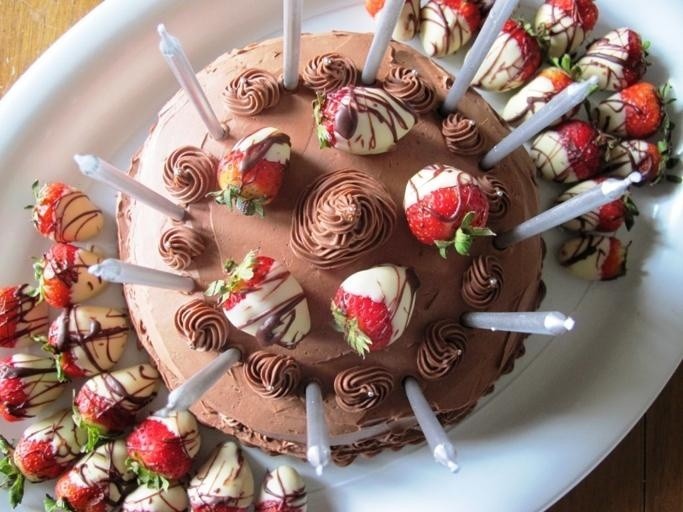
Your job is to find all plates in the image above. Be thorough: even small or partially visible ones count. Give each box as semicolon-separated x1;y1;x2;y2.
0;0;681;512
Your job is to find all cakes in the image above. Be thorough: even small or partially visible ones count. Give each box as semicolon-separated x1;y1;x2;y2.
70;0;645;472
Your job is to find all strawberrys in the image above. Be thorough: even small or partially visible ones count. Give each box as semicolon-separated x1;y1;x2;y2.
361;1;680;283
0;179;310;512
